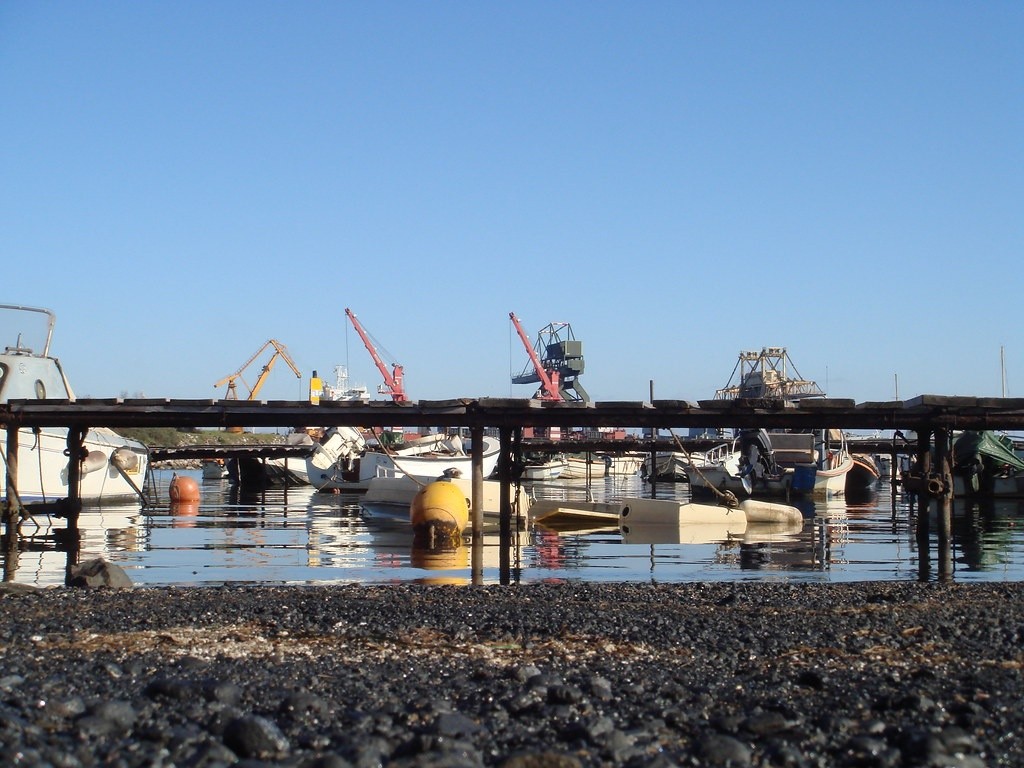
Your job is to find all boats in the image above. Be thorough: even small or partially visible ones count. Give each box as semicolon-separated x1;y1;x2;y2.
216;345;1024;582
0;304;151;501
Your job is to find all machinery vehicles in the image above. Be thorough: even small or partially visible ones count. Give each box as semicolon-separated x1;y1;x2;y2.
212;339;302;435
477;304;631;441
337;306;425;444
